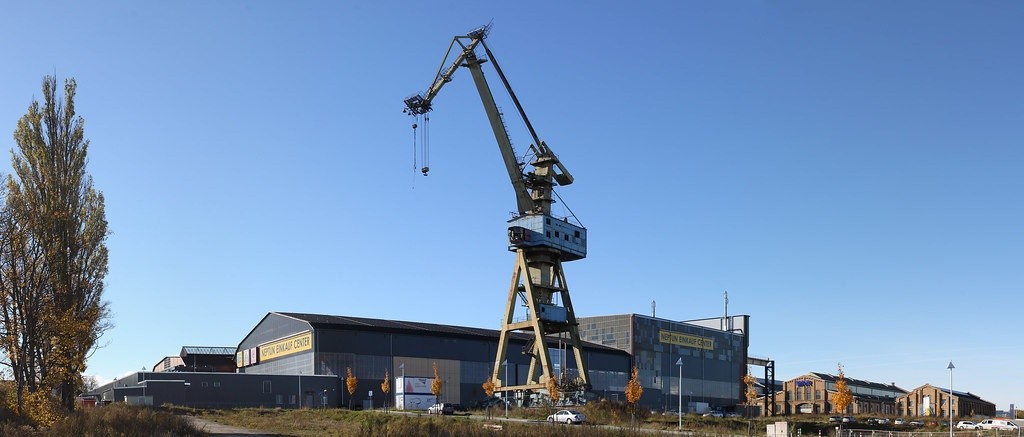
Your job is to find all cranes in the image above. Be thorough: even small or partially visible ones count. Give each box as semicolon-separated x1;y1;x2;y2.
403;14;594;396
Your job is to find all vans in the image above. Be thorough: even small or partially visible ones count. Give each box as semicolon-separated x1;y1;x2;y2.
991;420;1019;432
978;419;993;430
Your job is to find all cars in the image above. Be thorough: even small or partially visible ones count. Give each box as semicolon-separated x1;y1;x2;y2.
428;403;454;415
910;419;923;427
957;421;980;431
867;417;878;425
547;409;586;424
877;418;887;425
894;418;906;425
940;422;949;428
842;416;856;423
829;416;842;423
929;422;939;427
